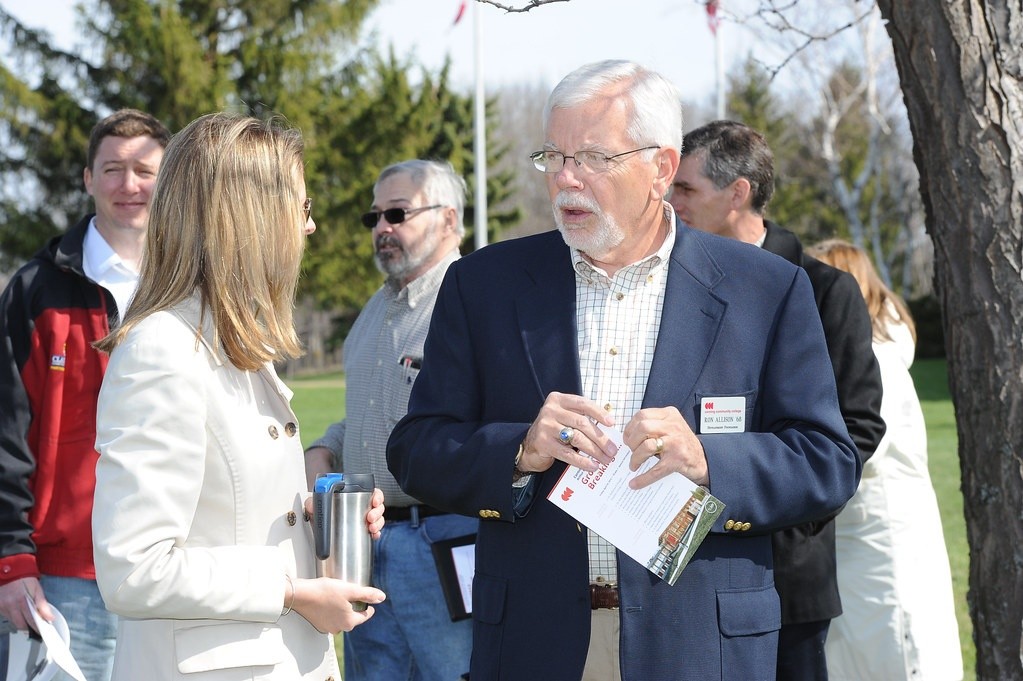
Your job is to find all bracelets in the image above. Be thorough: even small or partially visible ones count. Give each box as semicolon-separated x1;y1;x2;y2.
281;573;294;617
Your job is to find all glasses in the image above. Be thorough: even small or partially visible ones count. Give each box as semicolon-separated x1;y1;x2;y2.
361;204;445;227
303;197;312;222
528;146;660;172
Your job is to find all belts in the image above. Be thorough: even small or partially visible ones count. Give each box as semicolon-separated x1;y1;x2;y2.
382;504;449;522
590;584;619;610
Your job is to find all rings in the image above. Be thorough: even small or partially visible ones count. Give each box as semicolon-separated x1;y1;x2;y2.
652;436;665;454
559;426;575;445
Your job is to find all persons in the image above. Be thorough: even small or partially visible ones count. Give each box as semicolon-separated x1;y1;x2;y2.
92;108;386;681
387;61;861;681
797;235;966;681
303;156;479;681
670;120;887;681
0;107;177;681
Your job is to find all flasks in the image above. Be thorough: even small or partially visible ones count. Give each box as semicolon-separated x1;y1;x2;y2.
313;472;375;613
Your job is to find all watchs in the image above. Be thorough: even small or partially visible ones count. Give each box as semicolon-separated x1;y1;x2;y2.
514;439;537;478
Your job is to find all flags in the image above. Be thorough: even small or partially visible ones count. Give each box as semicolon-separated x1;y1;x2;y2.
704;0;723;34
449;1;473;29
